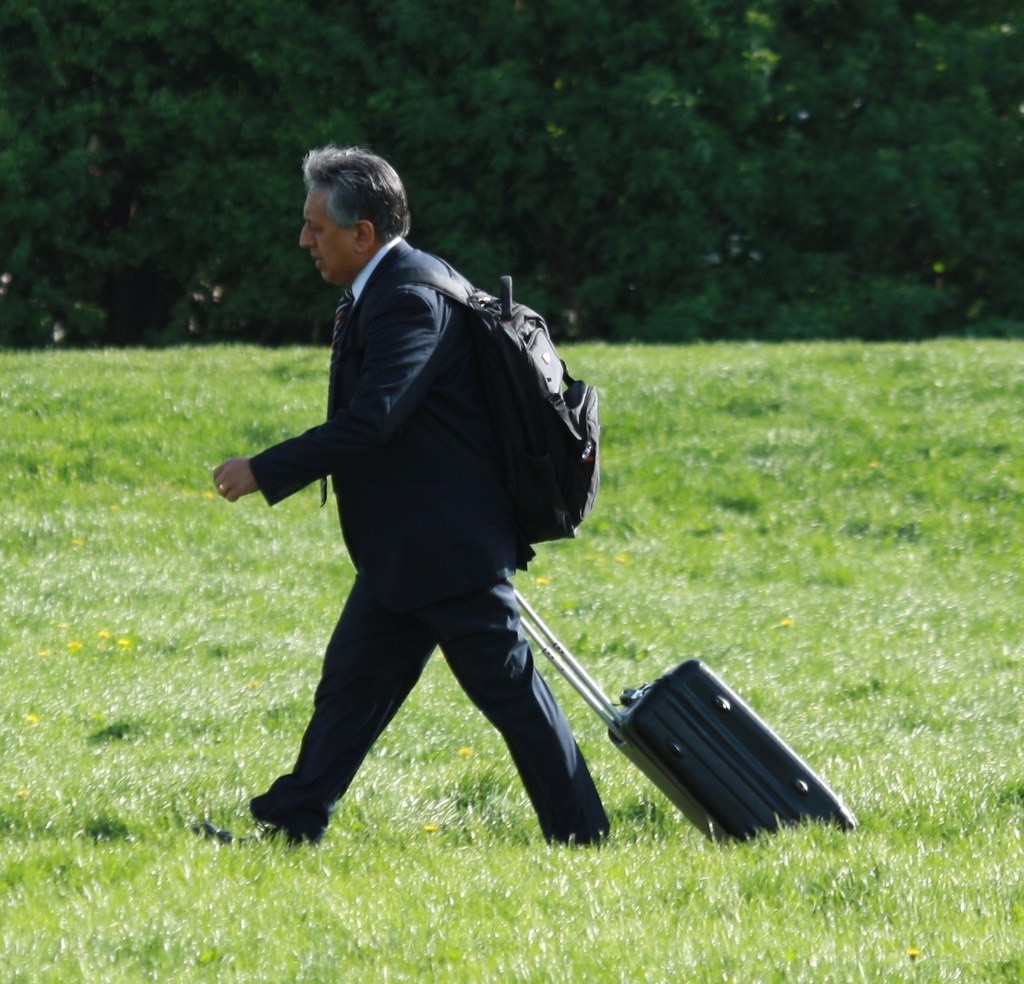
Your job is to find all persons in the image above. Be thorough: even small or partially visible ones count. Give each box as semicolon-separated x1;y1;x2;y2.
193;147;610;851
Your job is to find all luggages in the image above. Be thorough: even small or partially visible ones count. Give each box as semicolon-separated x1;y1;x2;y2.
499;573;856;848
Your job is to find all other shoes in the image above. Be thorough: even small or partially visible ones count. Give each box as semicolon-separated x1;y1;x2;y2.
192;814;284;851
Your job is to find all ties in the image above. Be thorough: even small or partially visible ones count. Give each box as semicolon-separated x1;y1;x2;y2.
316;289;354;508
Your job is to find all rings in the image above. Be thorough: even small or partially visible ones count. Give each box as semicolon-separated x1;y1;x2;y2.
219;483;227;493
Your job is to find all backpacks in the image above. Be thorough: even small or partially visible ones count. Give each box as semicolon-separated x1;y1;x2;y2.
349;255;603;543
